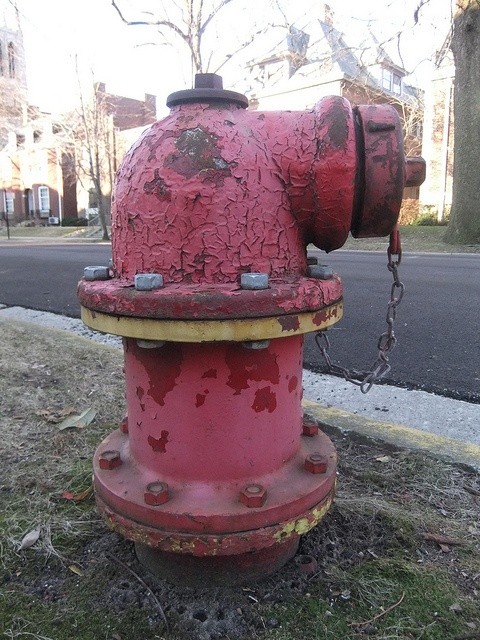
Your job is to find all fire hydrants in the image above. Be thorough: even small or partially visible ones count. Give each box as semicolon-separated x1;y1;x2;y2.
74;71;427;588
